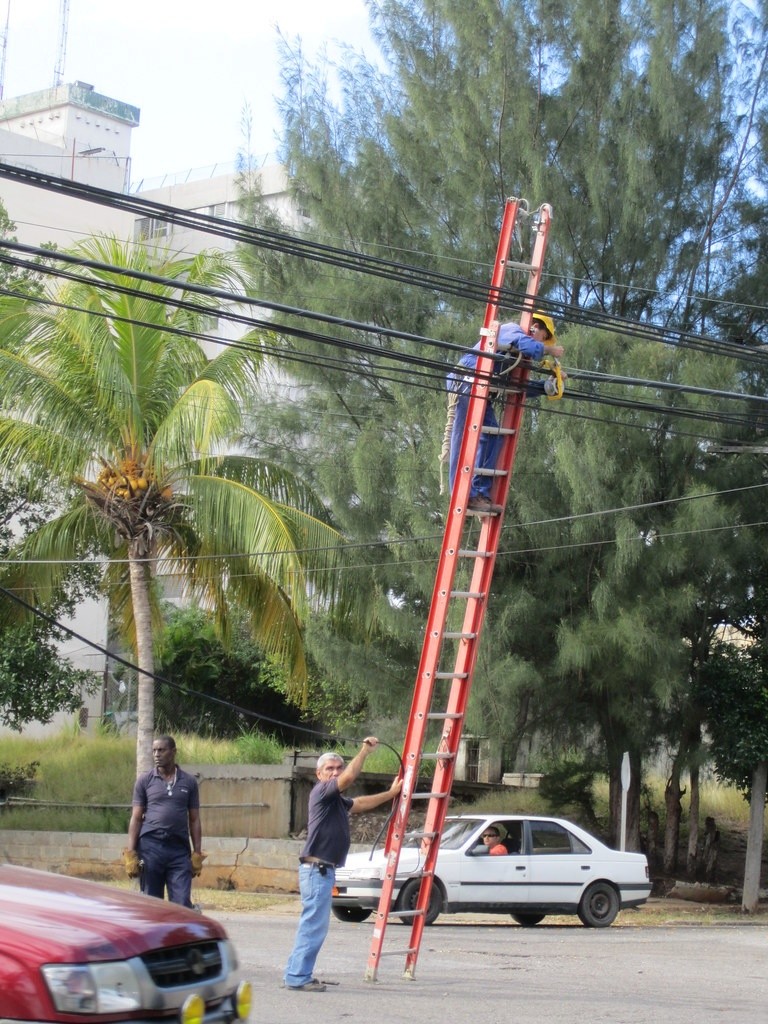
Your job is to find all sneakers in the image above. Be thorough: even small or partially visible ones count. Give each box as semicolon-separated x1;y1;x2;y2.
282;978;327;992
467;497;504;514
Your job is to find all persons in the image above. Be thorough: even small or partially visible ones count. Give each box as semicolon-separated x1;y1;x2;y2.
445;308;568;513
480;826;509;855
119;734;209;911
279;736;404;992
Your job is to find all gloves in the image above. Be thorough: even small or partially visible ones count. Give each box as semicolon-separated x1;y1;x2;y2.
125;847;141;879
191;851;208;878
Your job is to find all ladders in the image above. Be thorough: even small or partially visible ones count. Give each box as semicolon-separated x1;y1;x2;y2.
362;195;555;982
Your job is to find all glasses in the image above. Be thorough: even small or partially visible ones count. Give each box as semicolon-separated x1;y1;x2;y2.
482;833;498;838
166;783;173;797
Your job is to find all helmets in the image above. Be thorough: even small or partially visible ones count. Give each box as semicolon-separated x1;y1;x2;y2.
532;309;557;346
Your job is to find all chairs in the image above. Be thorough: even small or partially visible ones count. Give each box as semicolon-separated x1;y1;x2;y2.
501;826;521;852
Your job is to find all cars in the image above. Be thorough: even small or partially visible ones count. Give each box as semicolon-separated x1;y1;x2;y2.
1;862;253;1024
331;815;654;928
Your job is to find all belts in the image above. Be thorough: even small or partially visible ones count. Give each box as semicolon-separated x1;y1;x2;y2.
298;856;334;868
